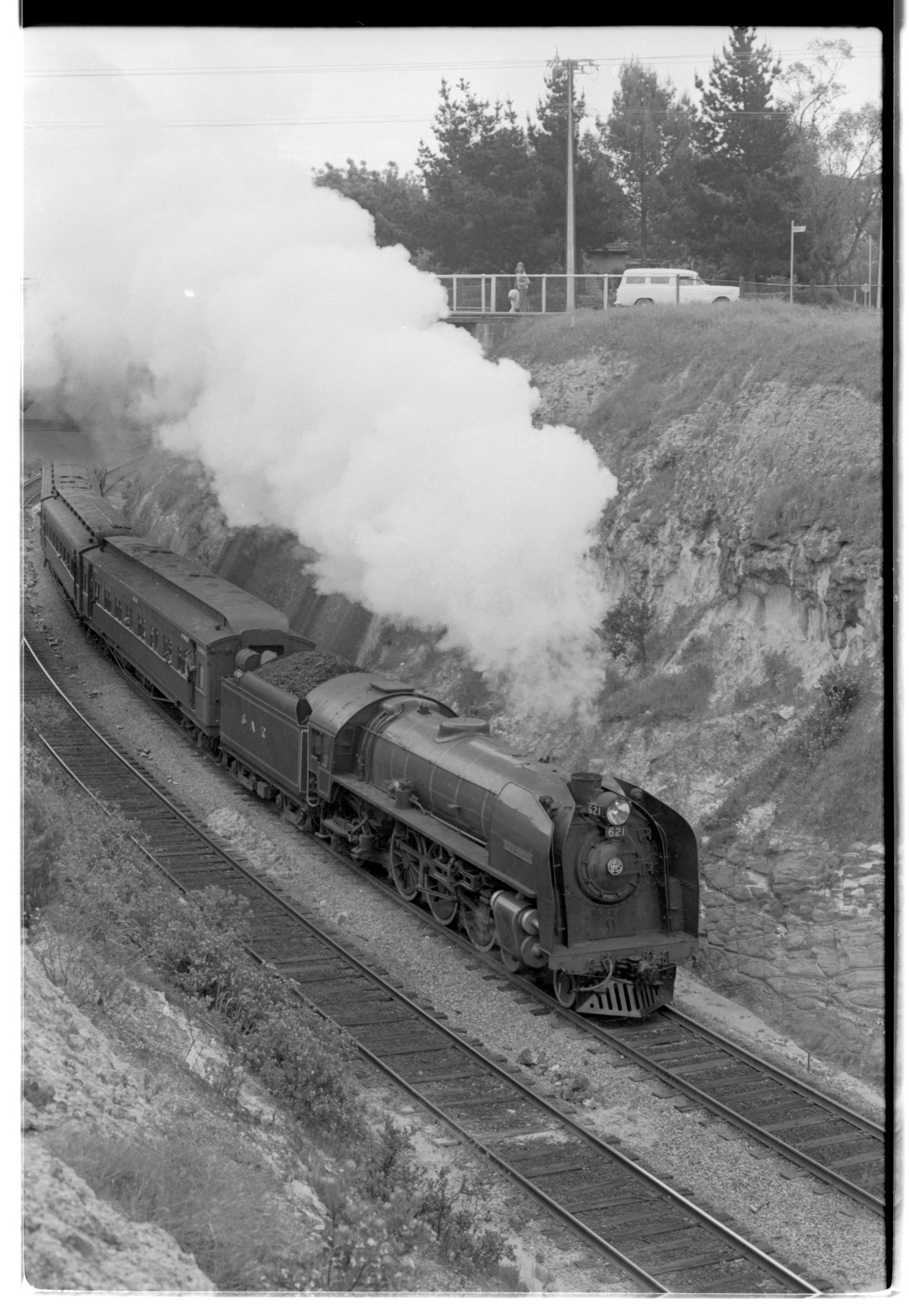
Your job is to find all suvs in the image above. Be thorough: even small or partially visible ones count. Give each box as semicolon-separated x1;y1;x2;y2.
614;268;740;310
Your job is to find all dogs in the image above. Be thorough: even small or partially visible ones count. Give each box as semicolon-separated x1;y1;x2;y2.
508;289;533;312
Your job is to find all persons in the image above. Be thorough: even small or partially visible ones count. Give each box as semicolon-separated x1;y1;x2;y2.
515;262;530;312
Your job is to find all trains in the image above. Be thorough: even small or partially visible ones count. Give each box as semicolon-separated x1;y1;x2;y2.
39;460;707;1025
